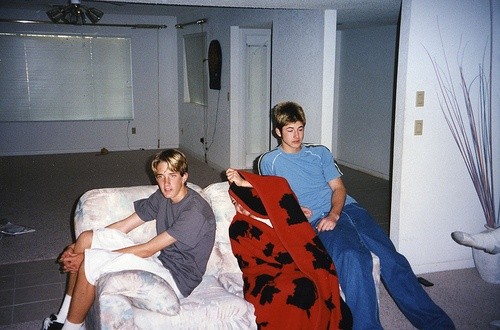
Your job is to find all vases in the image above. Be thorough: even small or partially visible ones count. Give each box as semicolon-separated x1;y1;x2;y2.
472;225;500;285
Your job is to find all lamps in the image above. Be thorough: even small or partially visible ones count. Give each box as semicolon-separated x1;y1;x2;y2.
47;0;104;25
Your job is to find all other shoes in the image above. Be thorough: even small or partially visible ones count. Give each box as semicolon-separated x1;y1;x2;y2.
42;314;64;330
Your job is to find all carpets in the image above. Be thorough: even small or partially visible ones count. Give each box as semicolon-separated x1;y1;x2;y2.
0;149;229;265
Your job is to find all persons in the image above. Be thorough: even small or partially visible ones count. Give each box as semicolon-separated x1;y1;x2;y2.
226;168;355;330
42;149;217;330
259;101;456;330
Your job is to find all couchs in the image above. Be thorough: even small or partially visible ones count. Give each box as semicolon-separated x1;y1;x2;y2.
73;182;380;330
